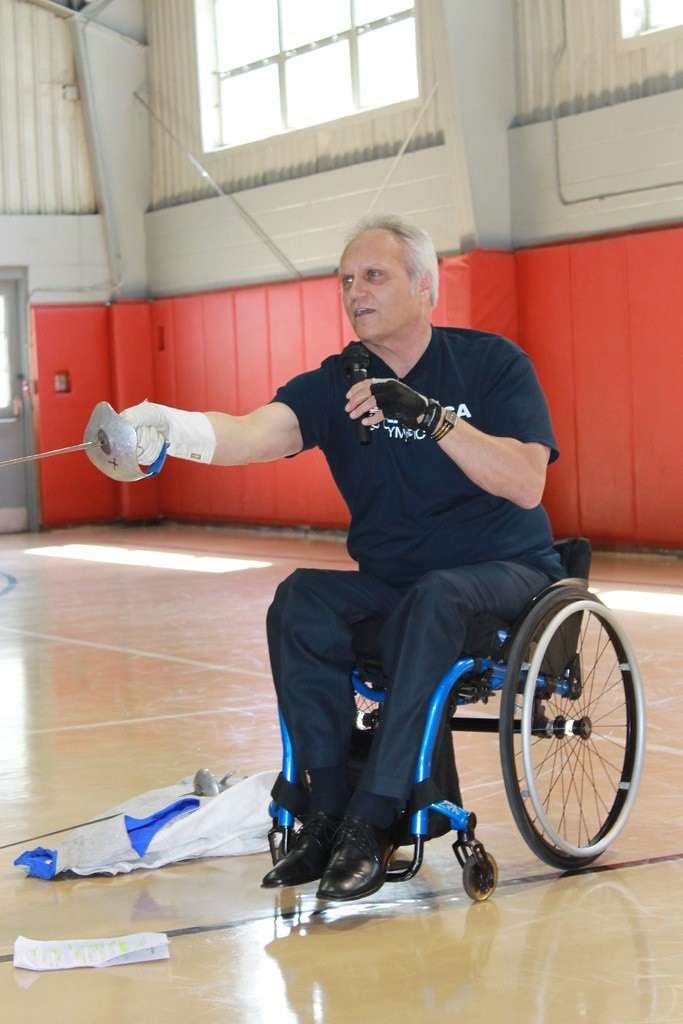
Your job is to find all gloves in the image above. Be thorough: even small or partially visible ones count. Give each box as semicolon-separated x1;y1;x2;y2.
119;403;169;465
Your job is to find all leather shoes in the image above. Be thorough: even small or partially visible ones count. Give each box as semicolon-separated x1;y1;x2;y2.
316;810;409;901
260;809;339;888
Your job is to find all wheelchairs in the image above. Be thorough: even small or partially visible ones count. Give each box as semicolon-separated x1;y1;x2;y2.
266;534;646;903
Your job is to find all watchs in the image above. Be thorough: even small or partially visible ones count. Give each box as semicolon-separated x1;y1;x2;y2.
431;408;458;442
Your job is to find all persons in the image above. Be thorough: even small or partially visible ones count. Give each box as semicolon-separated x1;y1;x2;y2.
120;211;566;901
